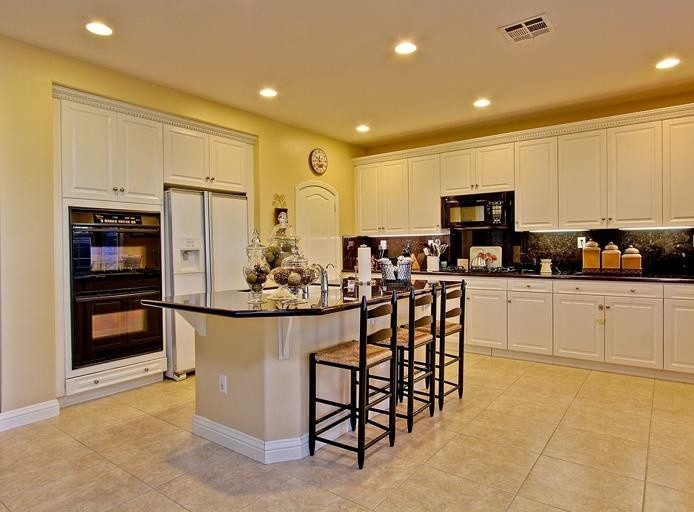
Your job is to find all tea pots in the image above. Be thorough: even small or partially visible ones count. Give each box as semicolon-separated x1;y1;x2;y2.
471;248;490;268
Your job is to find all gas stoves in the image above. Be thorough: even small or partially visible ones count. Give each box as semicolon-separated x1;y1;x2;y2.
441;264;523;276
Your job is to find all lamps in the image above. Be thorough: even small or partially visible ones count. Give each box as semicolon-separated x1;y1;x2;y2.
309;149;328;175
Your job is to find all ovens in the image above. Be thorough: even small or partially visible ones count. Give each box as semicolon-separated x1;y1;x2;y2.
70;290;165;368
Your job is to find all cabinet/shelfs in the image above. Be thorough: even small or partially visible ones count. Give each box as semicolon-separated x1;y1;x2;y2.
557;118;662;229
662;115;693;229
553;279;663;371
353;158;407;239
60;99;163;205
662;282;694;376
515;135;558;232
343;271;507;351
163;124;247;194
406;152;448;237
440;142;515;192
508;277;553;357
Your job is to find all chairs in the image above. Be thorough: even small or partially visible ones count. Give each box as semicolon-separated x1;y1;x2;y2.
310;280;466;470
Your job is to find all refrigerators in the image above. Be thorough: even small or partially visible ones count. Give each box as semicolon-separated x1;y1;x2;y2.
165;186;250;381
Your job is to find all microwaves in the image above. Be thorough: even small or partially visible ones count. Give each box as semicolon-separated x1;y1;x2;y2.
441;197;507;227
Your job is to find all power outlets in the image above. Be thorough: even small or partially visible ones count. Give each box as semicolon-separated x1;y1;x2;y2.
577;237;586;248
218;374;228;393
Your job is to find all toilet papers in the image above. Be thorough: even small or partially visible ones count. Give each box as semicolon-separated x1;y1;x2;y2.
359;286;371;301
358;247;371;281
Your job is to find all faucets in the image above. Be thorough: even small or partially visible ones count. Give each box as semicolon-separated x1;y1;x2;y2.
326;263;342;278
